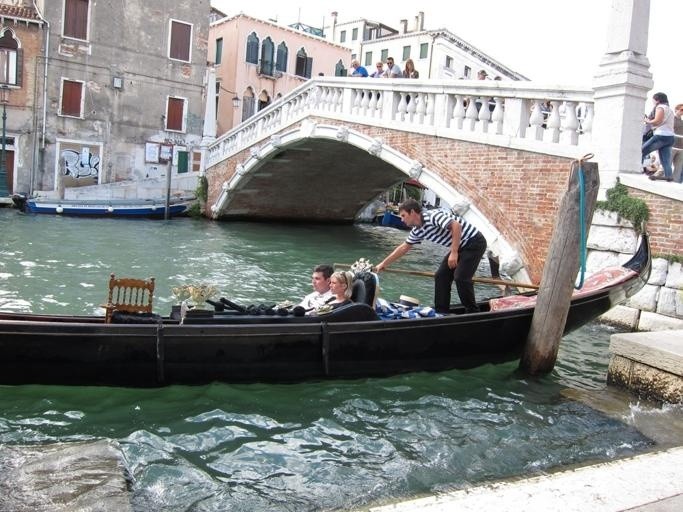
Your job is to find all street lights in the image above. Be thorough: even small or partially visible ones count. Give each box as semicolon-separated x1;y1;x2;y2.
0;82;9;197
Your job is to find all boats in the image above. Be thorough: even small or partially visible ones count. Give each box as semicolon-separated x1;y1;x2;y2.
10;193;187;219
374;203;411;230
0;219;653;389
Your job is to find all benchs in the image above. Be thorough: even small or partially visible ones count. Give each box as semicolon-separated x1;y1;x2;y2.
346;272;383;309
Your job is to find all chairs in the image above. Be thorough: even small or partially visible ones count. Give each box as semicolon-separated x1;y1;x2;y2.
99;272;155;323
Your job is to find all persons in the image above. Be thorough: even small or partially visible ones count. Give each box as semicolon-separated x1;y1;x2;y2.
402;59;419;113
324;271;353;310
351;59;368;77
319;73;324;76
297;265;336;315
379;57;402;112
473;70;552;129
642;92;683;184
375;199;487;314
369;62;384;78
